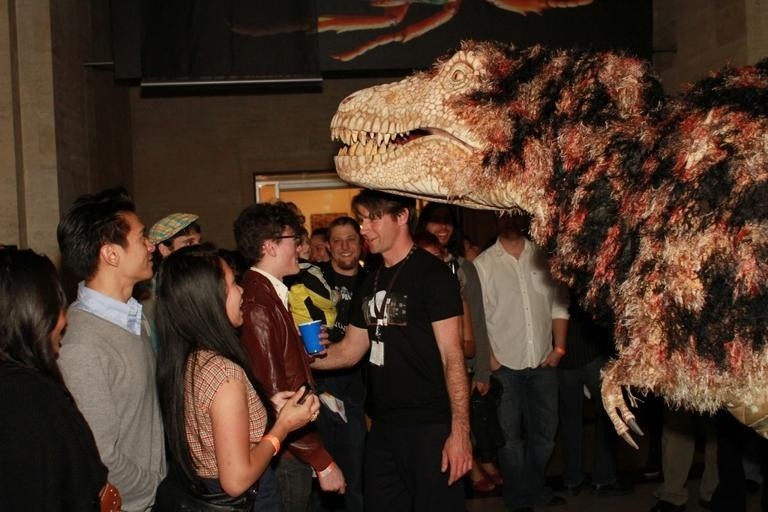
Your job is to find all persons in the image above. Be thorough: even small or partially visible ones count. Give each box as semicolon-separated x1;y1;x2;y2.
58;185;767;511
0;244;125;511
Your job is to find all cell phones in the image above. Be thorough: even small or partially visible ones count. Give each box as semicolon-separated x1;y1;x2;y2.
292;380;313;404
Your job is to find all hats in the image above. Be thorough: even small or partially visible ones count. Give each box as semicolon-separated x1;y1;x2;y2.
148;212;200;245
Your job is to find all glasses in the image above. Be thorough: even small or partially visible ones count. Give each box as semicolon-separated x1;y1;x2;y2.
260;232;302;246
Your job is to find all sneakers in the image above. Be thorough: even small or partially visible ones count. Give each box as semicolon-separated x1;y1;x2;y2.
649;499;688;512
698;497;712;509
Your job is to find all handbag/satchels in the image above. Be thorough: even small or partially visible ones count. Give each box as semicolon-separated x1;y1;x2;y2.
174;484;255;511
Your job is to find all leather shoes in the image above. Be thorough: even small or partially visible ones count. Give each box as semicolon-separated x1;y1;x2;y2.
463;469;505;493
542;477;635;509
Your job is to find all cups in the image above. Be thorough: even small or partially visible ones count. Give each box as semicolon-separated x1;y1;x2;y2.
298;319;325;355
